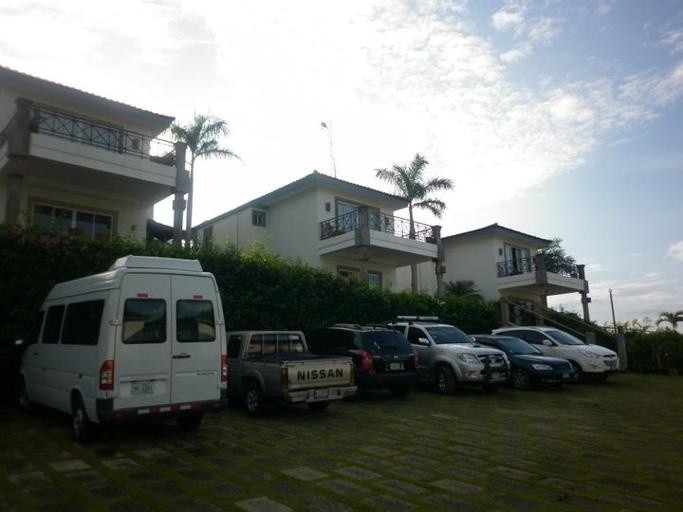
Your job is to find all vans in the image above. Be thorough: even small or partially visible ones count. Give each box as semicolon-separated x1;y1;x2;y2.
15;256;228;443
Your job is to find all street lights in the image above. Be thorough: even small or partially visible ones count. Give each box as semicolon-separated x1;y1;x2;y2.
321;122;336;178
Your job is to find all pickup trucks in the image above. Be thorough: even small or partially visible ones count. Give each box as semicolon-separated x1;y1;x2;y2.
227;325;358;418
